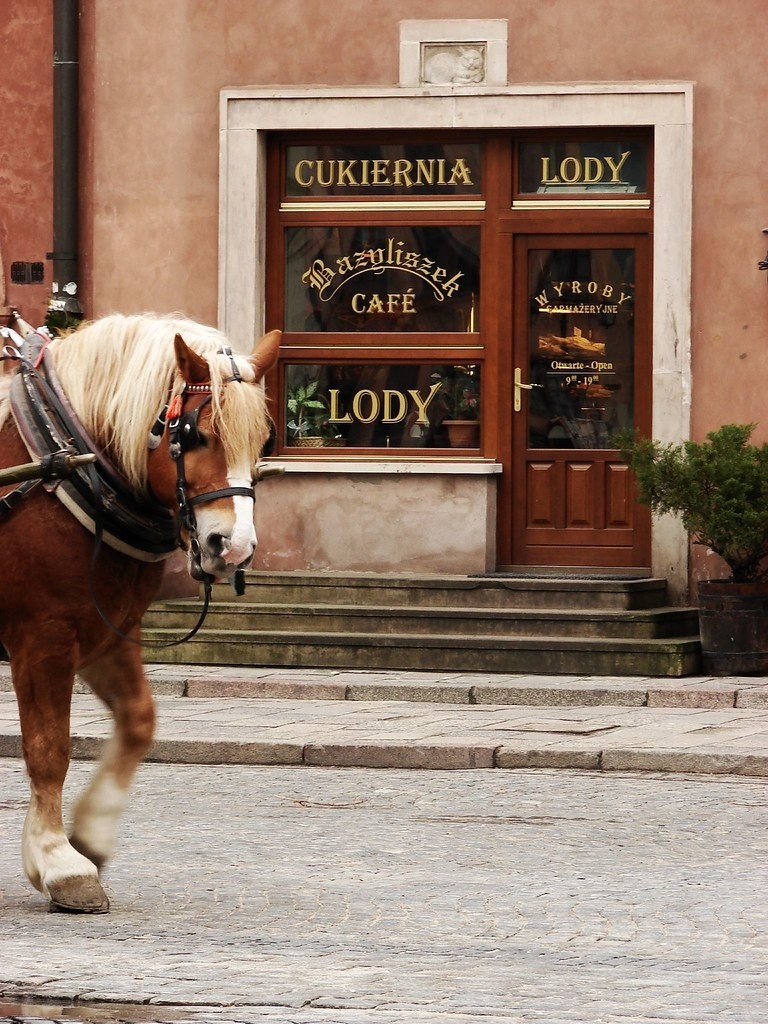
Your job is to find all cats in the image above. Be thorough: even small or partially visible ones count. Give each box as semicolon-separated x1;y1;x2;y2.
424;46;484;84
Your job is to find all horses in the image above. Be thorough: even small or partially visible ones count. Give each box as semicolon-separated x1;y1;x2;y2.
0;312;283;914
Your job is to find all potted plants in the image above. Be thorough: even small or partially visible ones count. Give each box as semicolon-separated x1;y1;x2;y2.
603;421;768;676
287;380;346;447
436;370;480;447
